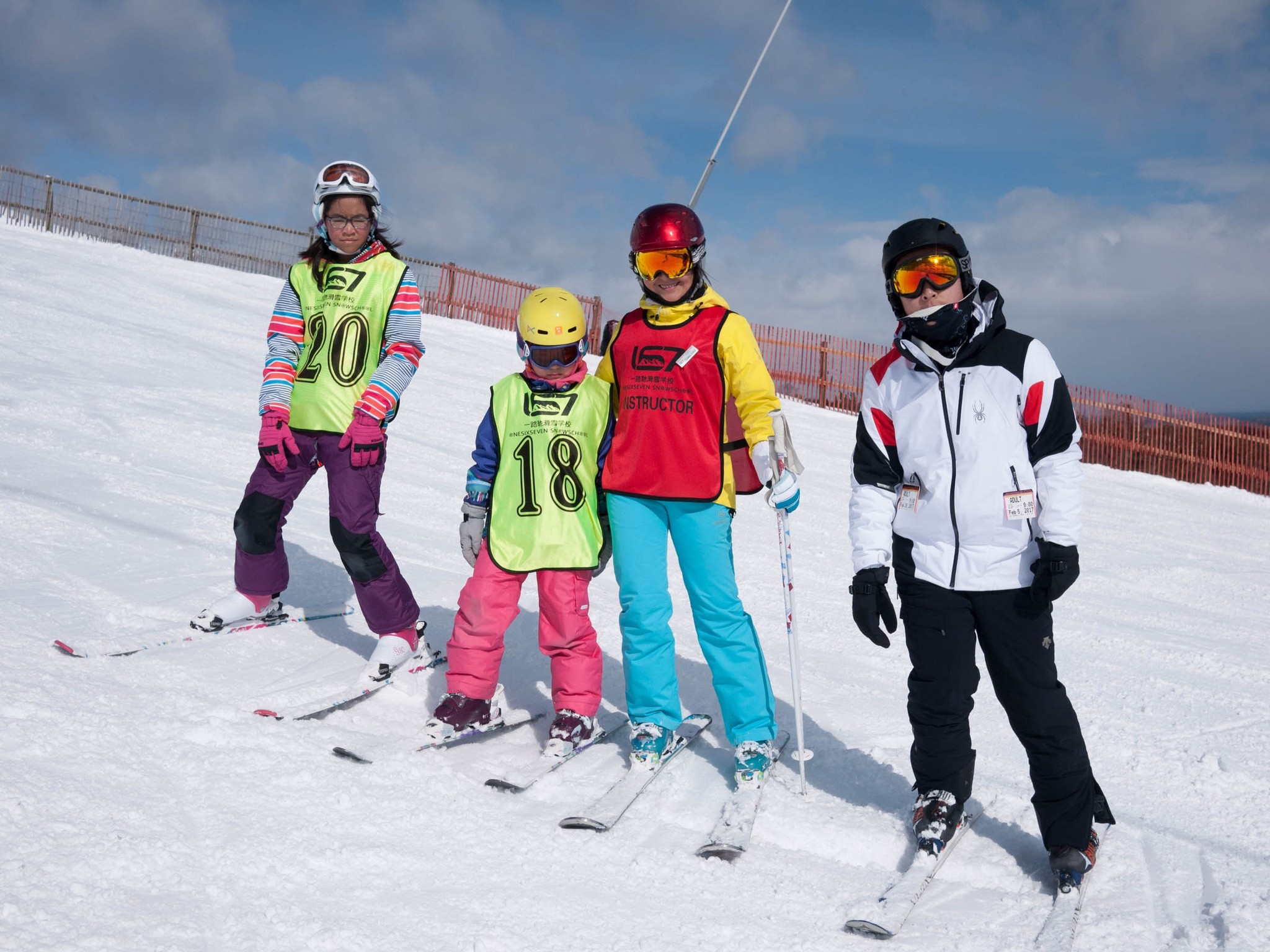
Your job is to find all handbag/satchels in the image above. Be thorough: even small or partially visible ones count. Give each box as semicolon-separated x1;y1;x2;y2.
591;515;612;577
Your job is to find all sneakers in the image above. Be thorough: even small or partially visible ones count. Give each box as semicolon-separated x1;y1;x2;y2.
433;693;492;732
368;621;418;668
910;789;963;846
734;739;771;772
209;589;272;623
629;723;673;760
549;710;594;744
1049;828;1097;878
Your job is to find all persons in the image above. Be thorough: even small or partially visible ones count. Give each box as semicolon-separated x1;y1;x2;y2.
189;160;426;681
848;218;1113;884
433;286;618;757
594;203;803;786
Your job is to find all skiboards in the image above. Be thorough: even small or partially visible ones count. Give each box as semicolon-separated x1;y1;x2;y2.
845;789;1110;951
332;711;628;794
49;601;448;724
559;714;787;860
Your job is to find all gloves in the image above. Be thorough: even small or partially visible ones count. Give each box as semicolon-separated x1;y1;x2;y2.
459;502;486;568
339;407;385;466
257;411;300;472
848;566;897;648
763;466;800;514
1027;537;1080;612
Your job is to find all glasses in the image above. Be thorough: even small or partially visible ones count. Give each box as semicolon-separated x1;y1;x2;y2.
892;252;961;297
325;214;370;229
529;343;579;370
635;247;692;282
318;160;374;191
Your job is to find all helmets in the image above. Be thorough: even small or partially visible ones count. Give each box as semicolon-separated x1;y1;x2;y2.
881;217;972;319
312;166;380;241
516;287;587;366
629;203;705;252
